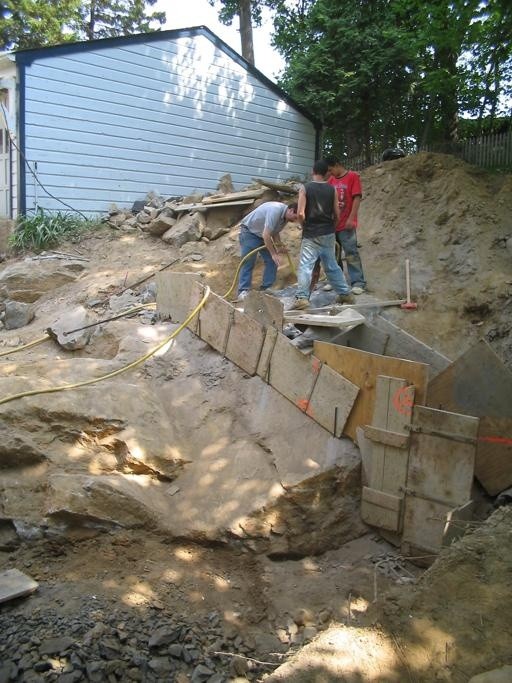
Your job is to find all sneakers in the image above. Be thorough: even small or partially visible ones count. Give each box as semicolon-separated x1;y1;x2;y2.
239;284;365;309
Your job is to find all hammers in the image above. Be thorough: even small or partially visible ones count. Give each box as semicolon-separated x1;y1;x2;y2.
400;259;417;309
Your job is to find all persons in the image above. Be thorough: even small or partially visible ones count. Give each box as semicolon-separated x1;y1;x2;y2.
236;199;298;303
292;159;357;310
322;156;366;295
